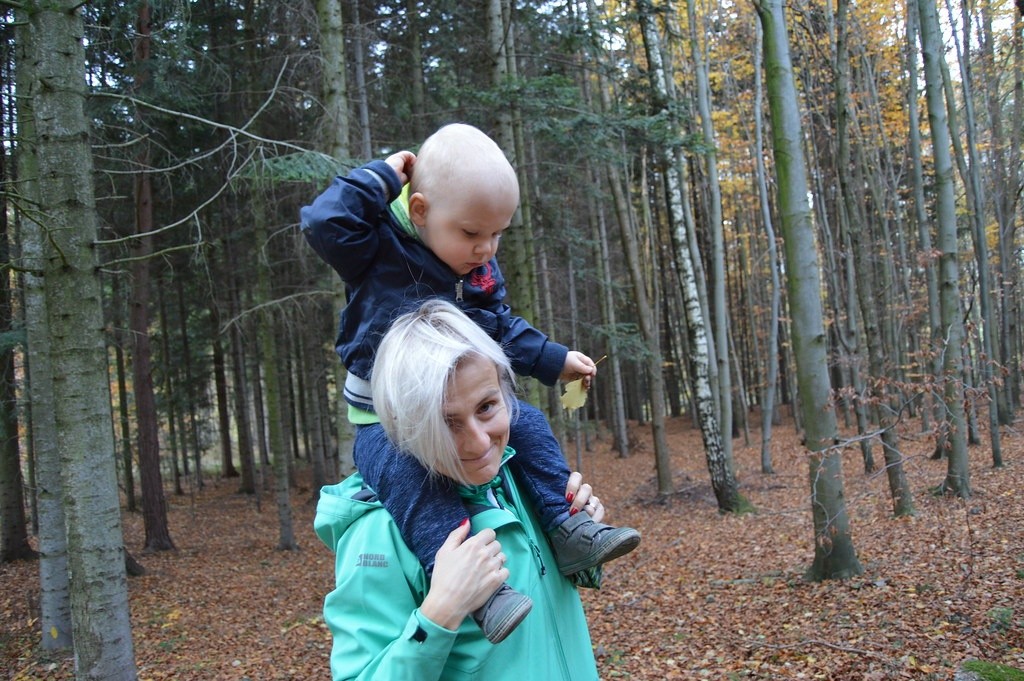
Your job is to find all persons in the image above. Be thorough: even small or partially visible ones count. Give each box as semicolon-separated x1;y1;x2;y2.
299;122;643;646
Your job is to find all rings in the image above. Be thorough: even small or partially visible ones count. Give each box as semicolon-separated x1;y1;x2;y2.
495;555;504;565
313;299;600;681
585;501;598;511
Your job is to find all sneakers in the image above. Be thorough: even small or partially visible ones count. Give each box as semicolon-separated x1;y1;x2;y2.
472;582;534;644
549;510;641;575
